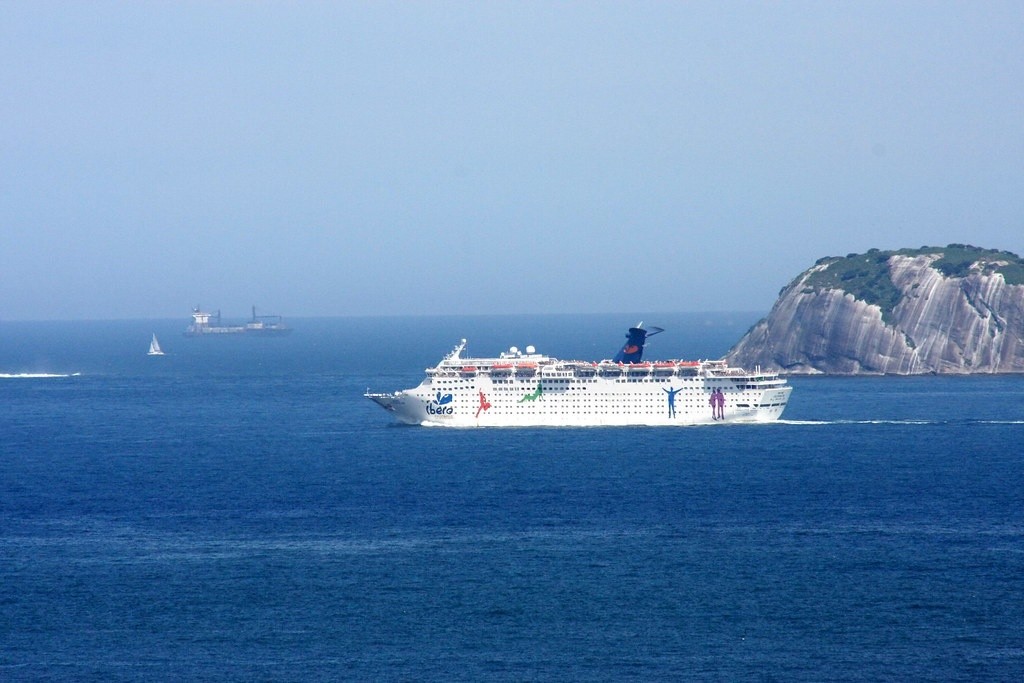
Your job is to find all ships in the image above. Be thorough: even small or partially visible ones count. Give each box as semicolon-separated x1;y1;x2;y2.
363;318;793;430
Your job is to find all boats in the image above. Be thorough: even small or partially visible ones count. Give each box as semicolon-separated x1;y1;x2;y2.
181;304;293;337
628;360;652;372
653;360;677;371
461;365;479;373
491;360;515;372
679;360;699;368
515;360;538;371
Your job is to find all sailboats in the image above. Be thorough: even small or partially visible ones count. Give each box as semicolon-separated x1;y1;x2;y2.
146;331;165;356
